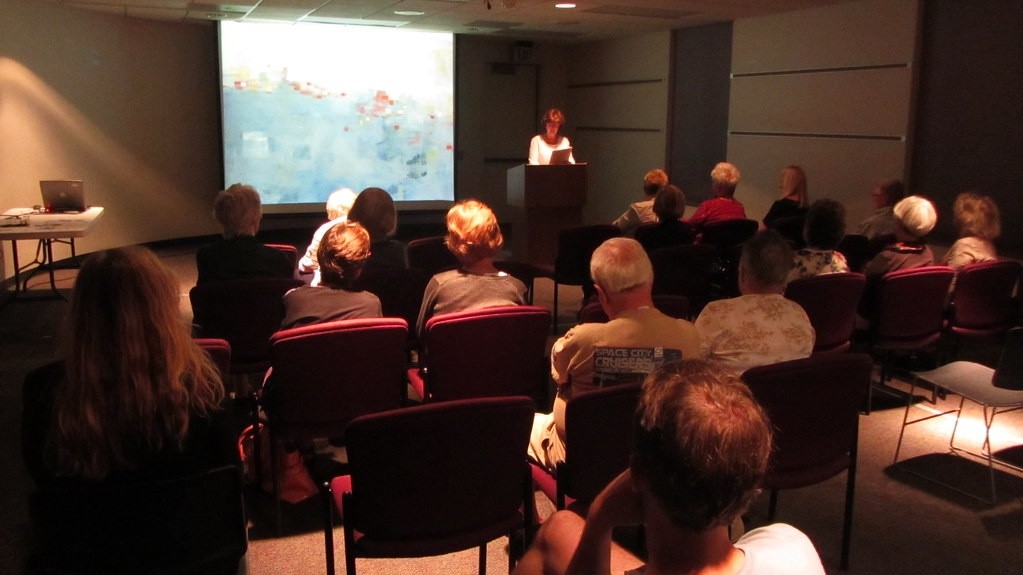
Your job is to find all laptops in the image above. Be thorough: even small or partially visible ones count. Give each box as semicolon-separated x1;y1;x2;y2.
549;147;572;166
39;180;92;212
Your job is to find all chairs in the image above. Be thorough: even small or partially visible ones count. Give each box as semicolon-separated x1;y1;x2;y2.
526;214;804;319
741;338;860;572
530;374;647;517
780;271;866;352
265;244;313;292
861;264;954;415
319;396;538;575
406;236;447;276
236;315;422;542
406;307;555;440
190;337;232;408
492;258;535;308
933;259;1023;394
893;323;1023;506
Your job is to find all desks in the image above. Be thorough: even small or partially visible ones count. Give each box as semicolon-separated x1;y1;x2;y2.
0;205;105;303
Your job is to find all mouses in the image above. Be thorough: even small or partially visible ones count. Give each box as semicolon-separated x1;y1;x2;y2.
33;205;41;210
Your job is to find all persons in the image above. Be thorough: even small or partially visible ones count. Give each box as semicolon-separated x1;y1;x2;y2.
784;181;1003;292
611;161;809;249
189;185;529;493
22;247;247;575
529;107;578;166
510;227;830;575
